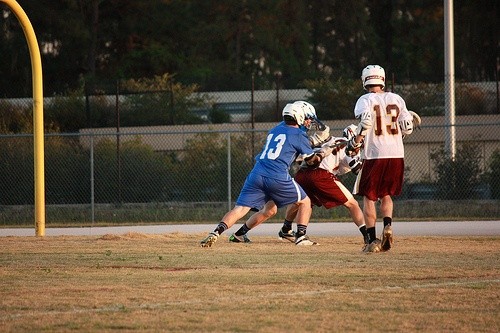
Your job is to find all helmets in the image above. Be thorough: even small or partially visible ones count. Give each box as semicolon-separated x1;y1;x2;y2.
282;103;305;126
344;125;359;140
293;100;316;120
361;65;386;91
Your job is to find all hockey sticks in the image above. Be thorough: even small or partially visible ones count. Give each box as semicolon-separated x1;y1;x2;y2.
313;140;349;153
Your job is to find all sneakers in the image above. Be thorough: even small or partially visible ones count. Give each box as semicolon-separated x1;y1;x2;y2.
201;231;219;248
229;232;250;243
363;239;380;253
381;226;392;251
279;228;297;243
295;235;318;246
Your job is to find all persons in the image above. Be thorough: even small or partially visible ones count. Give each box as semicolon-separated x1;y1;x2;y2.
281;123;366;242
200;101;335;246
345;64;413;253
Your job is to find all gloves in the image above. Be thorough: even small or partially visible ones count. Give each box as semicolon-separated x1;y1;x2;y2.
317;137;337;158
310;125;330;145
345;137;362;158
